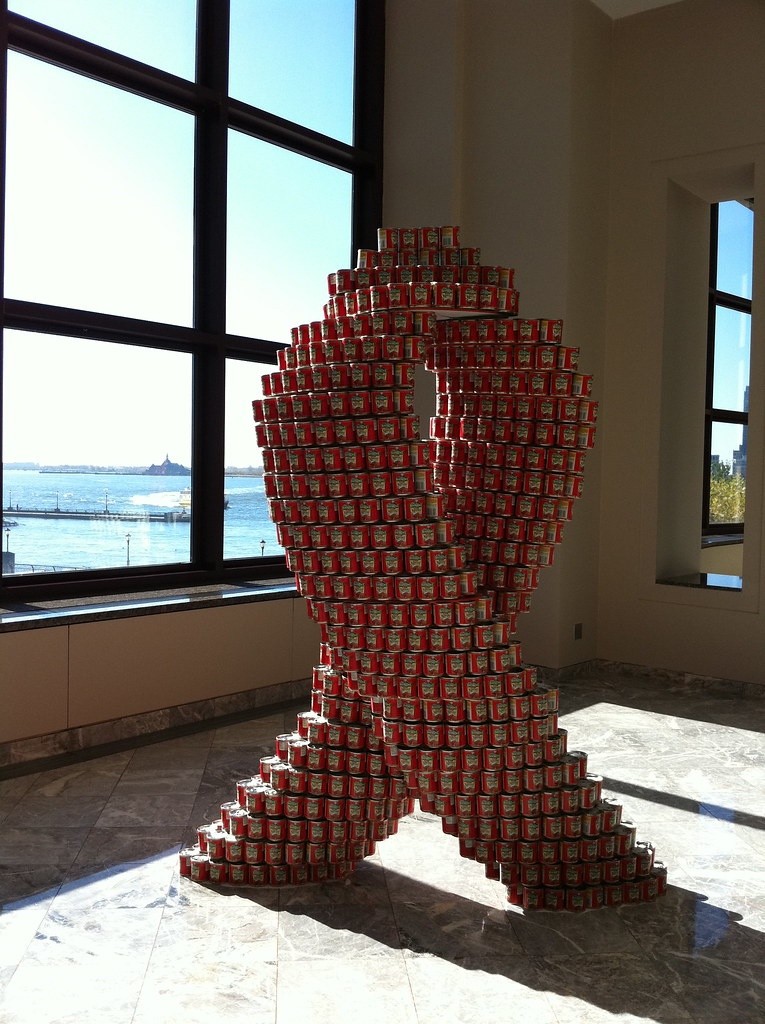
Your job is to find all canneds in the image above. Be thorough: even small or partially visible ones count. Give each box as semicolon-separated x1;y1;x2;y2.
180;226;667;912
